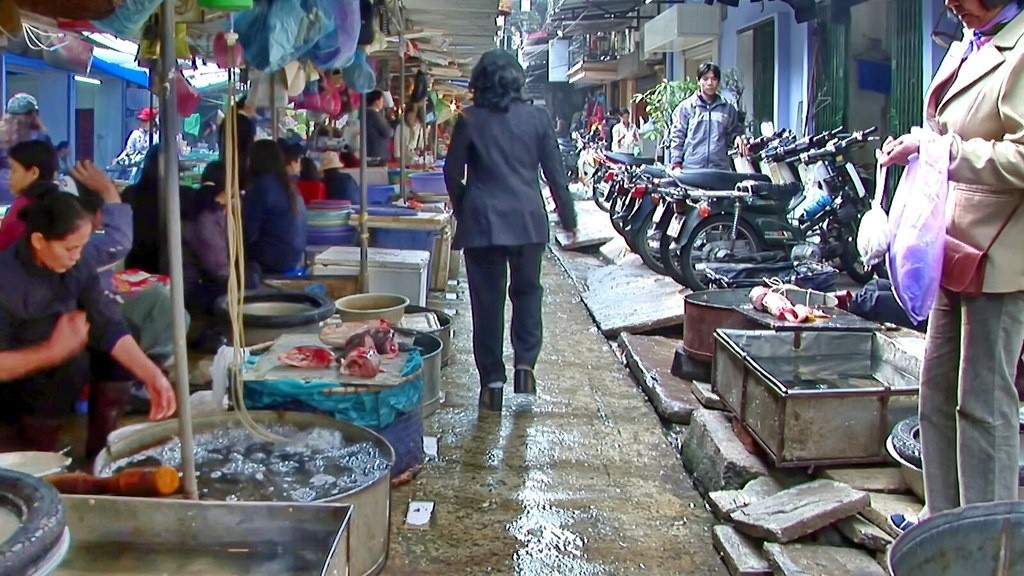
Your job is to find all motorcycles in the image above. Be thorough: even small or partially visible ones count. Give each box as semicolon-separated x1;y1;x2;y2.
538;113;902;295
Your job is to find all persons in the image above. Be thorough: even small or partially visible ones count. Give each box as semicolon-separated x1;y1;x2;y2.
443;50;579;411
554;113;570;138
611;108;639;153
670;63;748;171
0;90;426;454
875;0;1024;534
604;108;621;152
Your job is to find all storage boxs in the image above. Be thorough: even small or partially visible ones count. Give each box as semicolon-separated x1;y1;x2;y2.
299;213;454;307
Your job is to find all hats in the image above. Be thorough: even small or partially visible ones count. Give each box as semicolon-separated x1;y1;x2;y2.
7;92;39;115
319;151;344;170
137;107;157;121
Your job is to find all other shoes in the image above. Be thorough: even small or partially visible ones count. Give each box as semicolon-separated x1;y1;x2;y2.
888;513;919;535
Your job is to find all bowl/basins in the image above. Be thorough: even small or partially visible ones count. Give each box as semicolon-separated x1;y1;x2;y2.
334;292;410;325
358;185;399;205
407;171;449;195
91;305;455;576
885;435;1023;506
886;499;1024;576
681;287;837;365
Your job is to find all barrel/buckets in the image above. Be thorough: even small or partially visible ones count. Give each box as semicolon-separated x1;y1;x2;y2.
0;450;72;480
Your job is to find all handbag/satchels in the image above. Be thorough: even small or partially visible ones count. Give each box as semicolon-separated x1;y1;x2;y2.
885;128;950;326
857;157;890;264
939;234;987;298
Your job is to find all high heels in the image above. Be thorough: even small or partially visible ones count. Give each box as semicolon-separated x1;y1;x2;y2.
479;385;503;412
514;369;536;398
